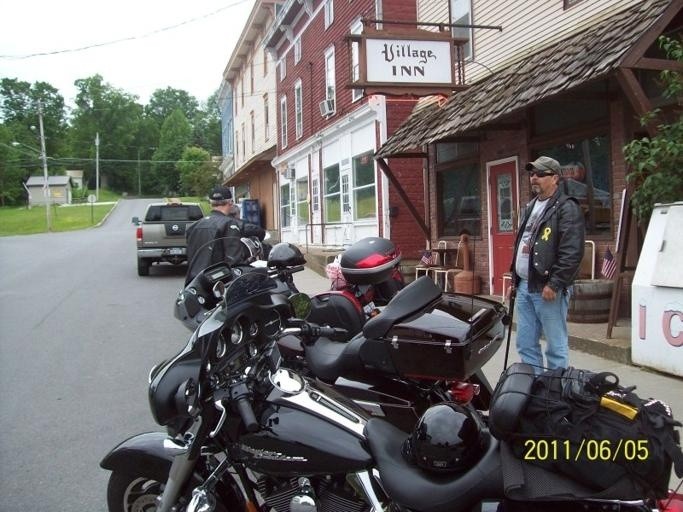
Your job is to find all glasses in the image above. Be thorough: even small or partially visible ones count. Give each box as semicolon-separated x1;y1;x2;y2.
530;170;554;177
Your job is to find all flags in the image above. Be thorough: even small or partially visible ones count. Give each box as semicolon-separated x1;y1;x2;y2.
601;247;616;279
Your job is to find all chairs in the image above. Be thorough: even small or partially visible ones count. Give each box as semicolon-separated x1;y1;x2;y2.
300;274;442;384
361;361;535;512
413;239;595;307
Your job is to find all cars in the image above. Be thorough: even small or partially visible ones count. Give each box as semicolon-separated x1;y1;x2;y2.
555;175;611;231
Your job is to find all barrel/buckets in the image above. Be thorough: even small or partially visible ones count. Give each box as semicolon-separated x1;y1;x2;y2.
566;280;615;323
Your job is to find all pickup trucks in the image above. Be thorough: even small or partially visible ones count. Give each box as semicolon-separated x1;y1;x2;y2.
130;202;209;276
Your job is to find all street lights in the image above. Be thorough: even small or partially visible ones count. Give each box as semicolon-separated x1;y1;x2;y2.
93;132;101;203
10;141;52;232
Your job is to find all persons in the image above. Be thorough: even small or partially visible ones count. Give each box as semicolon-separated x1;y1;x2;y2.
507;156;586;377
227;205;240;219
185;187;263;288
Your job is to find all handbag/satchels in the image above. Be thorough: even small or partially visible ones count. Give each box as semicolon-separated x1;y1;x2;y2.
512;364;683;500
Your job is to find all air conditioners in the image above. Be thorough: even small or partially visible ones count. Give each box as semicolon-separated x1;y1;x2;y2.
318;98;333;118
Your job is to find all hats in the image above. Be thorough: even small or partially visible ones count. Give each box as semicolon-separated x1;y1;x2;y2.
209;186;231;200
524;156;562;173
399;400;488;477
267;242;307;268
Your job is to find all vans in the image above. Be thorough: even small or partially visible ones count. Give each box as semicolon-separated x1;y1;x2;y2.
440;196;481;235
